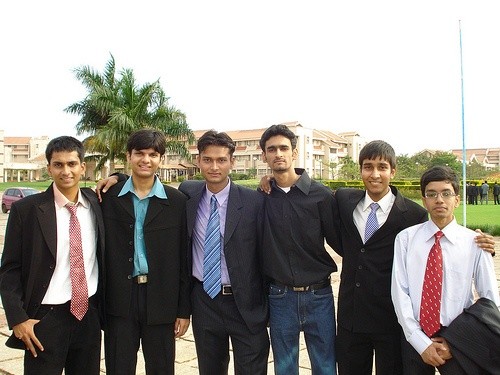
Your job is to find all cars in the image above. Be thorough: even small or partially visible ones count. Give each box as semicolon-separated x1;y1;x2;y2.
1;187;41;214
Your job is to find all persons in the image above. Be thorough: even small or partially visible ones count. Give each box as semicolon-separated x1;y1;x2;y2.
96;131;269;375
391;166;500;375
96;129;191;375
258;141;496;375
493;181;500;205
256;124;344;375
466;181;489;205
0;136;106;375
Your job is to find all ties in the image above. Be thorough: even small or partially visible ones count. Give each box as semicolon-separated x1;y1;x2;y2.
202;195;222;299
420;230;444;338
363;205;380;242
65;202;89;321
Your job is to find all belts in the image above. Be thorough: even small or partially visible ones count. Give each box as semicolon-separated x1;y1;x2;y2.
132;274;150;284
220;284;233;295
285;283;323;291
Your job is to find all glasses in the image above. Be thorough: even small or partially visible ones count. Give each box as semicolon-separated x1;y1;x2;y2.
425;191;457;200
